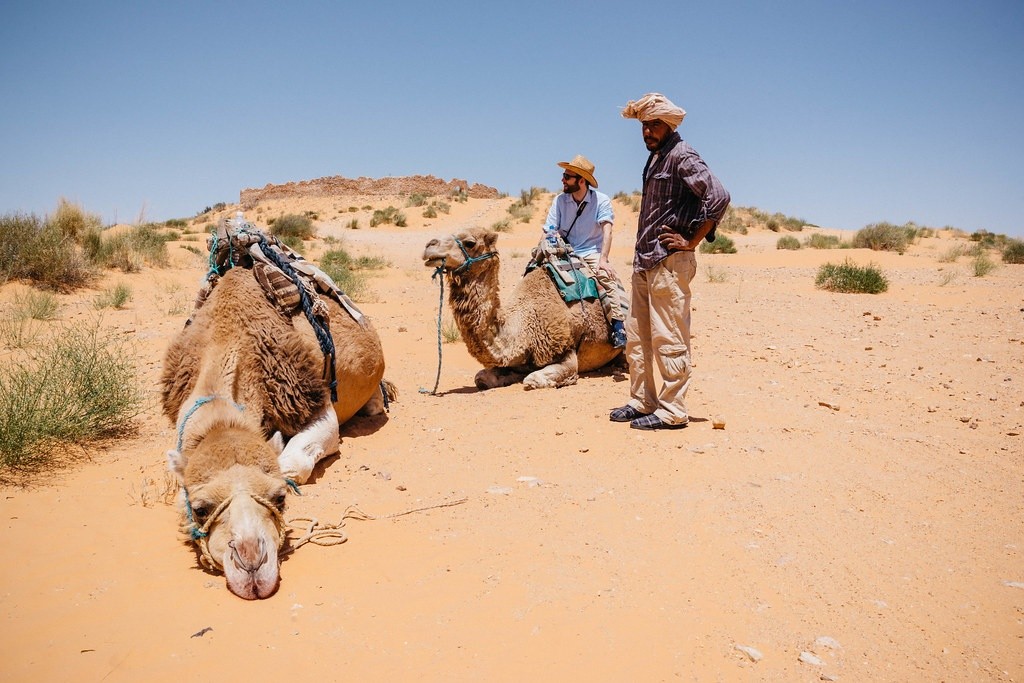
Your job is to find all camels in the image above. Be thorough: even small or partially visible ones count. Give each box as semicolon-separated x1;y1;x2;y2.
422;227;631;391
156;219;384;600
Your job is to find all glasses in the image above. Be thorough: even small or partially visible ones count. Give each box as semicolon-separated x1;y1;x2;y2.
563;172;578;180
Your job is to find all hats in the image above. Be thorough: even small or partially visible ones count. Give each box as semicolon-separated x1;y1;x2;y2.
557;156;598;188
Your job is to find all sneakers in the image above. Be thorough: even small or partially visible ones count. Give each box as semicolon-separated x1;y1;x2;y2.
612;329;627;350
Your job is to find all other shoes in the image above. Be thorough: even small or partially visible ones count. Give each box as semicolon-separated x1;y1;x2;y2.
630;413;686;430
610;405;652;422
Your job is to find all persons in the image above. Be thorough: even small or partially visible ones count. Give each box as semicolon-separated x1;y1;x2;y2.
609;93;731;430
523;155;627;348
